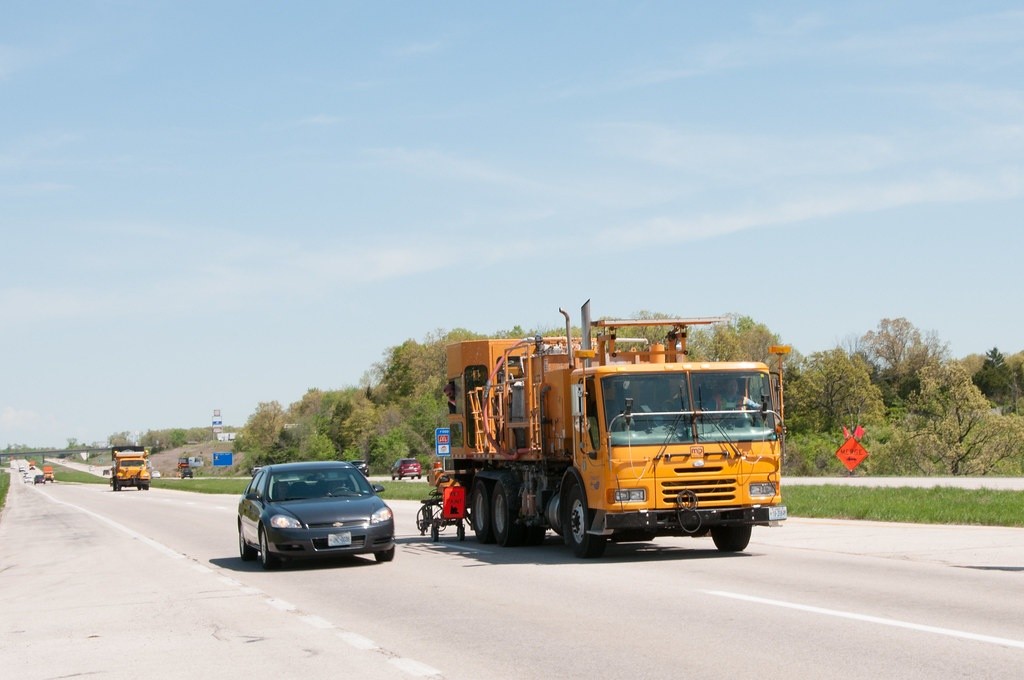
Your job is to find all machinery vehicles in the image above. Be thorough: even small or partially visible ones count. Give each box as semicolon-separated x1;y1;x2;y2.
446;299;793;557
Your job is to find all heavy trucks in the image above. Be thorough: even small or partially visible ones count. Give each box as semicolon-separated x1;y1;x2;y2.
112;446;151;491
43;466;54;482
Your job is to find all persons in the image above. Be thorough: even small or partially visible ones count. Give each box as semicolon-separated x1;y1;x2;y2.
701;377;768;423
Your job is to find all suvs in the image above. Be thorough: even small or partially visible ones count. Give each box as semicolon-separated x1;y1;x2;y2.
390;458;422;480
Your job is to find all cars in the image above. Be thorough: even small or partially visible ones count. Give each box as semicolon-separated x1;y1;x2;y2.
181;469;193;479
350;461;369;477
34;475;45;484
18;465;35;484
237;461;396;570
151;471;160;479
